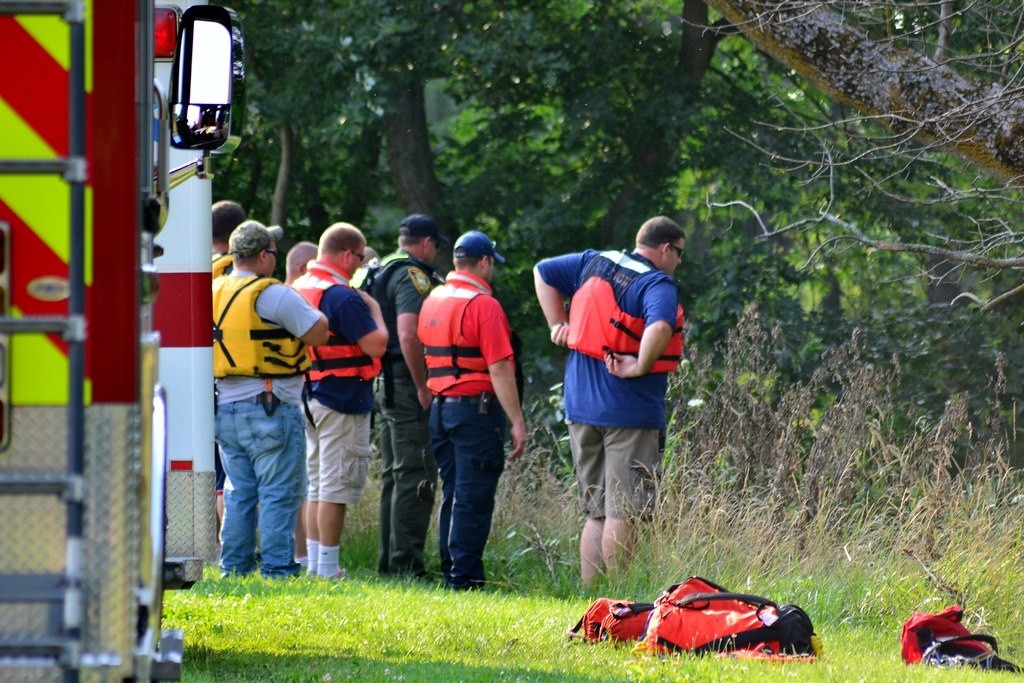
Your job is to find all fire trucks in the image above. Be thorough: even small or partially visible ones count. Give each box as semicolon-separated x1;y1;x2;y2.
0;1;250;683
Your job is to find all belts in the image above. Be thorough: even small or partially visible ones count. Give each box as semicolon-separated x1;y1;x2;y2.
240;391;280;404
432;396;501;406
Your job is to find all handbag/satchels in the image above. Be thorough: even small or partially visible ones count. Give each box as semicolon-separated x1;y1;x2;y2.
901;606;1020;673
569;599;650;646
642;576;819;663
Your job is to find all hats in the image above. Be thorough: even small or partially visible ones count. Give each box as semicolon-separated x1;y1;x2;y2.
454;231;505;263
228;221;283;258
400;214;452;244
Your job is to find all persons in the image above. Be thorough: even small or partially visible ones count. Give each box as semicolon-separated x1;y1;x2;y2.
532;215;685;585
212;200;527;593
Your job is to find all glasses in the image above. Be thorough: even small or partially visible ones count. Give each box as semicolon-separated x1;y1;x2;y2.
341;249;365;262
657;241;684;257
263;249;278;256
